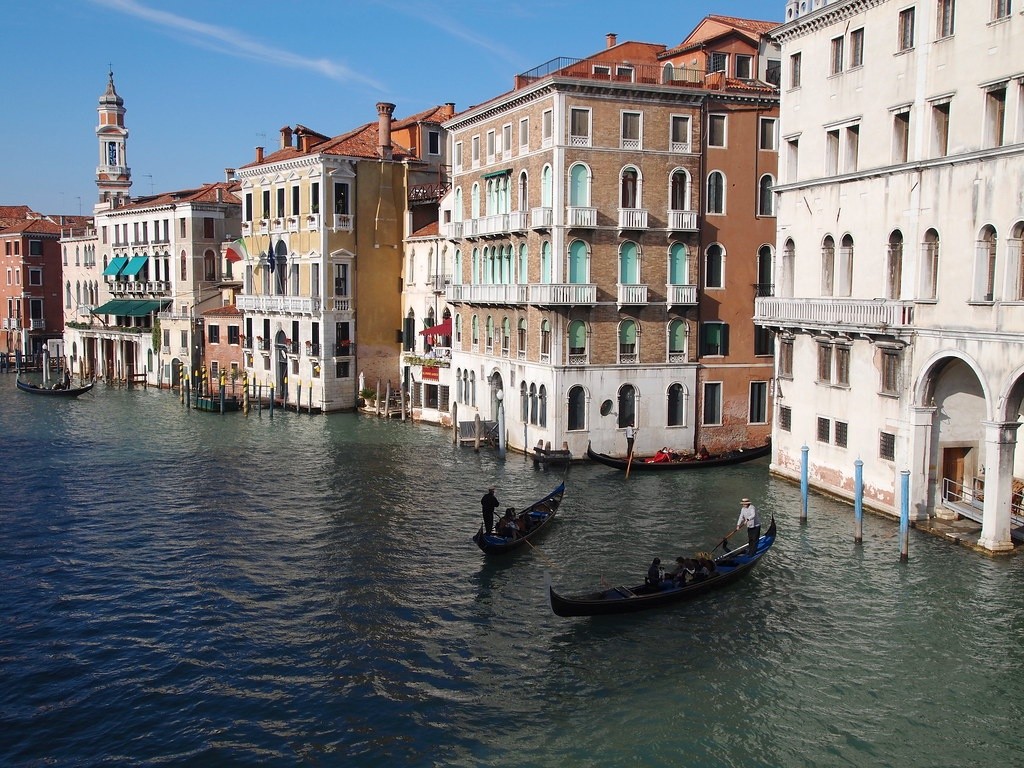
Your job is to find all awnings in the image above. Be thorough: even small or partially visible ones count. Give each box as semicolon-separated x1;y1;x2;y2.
419;318;452;337
90;256;172;318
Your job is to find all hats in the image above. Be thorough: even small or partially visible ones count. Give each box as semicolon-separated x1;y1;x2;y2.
488;486;495;489
740;498;751;504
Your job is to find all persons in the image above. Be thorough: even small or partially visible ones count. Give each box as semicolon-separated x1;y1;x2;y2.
626;422;637;458
498;508;524;537
28;381;65;391
646;445;710;462
648;556;716;589
480;488;499;537
737;498;761;558
65;368;71;390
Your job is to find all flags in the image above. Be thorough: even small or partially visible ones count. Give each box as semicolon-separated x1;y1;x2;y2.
226;238;249;265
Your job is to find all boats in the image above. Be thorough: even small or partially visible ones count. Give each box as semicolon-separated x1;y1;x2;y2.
550;510;777;618
15;378;94;395
473;480;565;555
586;439;772;472
